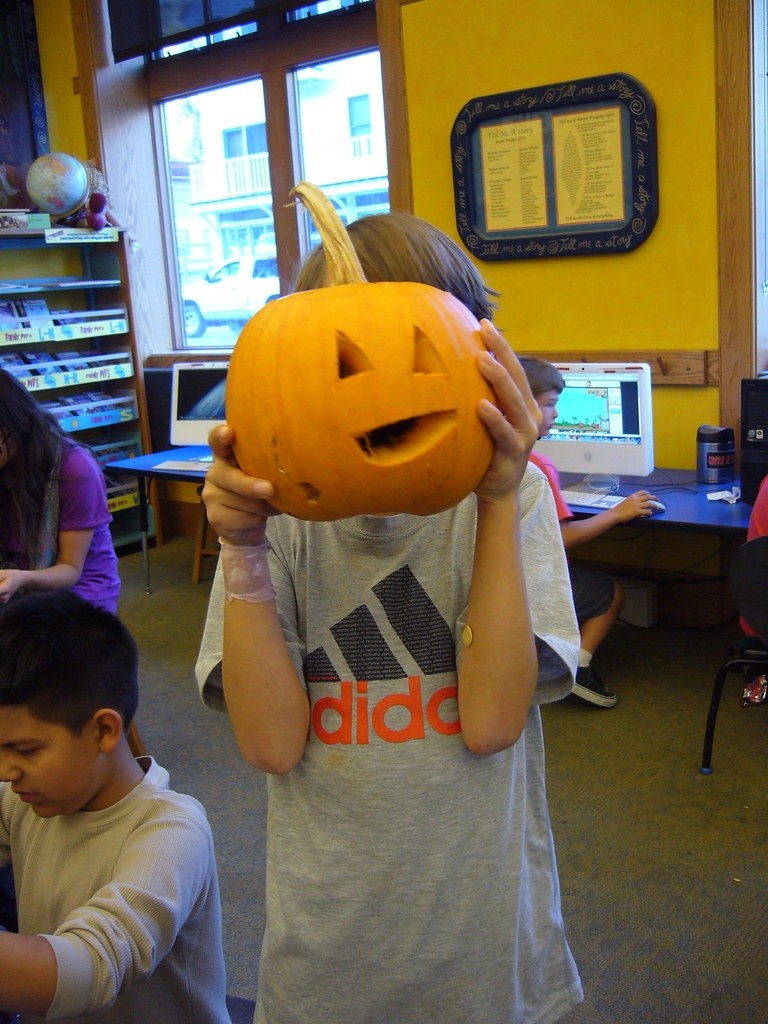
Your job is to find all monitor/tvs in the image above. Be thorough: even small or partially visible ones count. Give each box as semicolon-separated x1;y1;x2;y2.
170;361;231;462
530;362;655;495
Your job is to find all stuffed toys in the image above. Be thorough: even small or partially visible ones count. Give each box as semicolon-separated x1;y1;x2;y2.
64;166;133;247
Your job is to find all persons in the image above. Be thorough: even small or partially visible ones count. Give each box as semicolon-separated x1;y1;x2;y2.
0;368;122;616
0;216;19;228
1;584;231;1024
740;476;768;705
518;356;659;708
194;213;584;1024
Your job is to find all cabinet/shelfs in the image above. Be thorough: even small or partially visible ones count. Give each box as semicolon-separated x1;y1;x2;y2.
1;227;165;558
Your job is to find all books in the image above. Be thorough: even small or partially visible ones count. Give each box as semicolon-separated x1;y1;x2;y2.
82;434;133;498
38;390;122;421
0;280;121;289
0;351;114;378
0;299;83;331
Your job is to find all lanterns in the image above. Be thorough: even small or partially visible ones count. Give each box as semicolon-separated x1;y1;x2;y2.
225;179;497;523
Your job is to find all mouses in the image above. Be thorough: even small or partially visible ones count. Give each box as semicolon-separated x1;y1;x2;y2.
640;499;666;517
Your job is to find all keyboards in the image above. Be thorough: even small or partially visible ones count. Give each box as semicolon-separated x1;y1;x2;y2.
152;461;213;472
560;489;627;510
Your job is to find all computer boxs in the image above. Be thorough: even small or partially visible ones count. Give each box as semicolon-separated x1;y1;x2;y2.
741;374;768;505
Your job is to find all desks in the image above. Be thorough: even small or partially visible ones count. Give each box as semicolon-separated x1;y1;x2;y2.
106;447;752;597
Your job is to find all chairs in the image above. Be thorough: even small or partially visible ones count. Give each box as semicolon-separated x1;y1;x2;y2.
700;536;768;775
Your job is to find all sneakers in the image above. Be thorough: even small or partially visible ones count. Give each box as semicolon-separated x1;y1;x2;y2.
571;664;617;706
